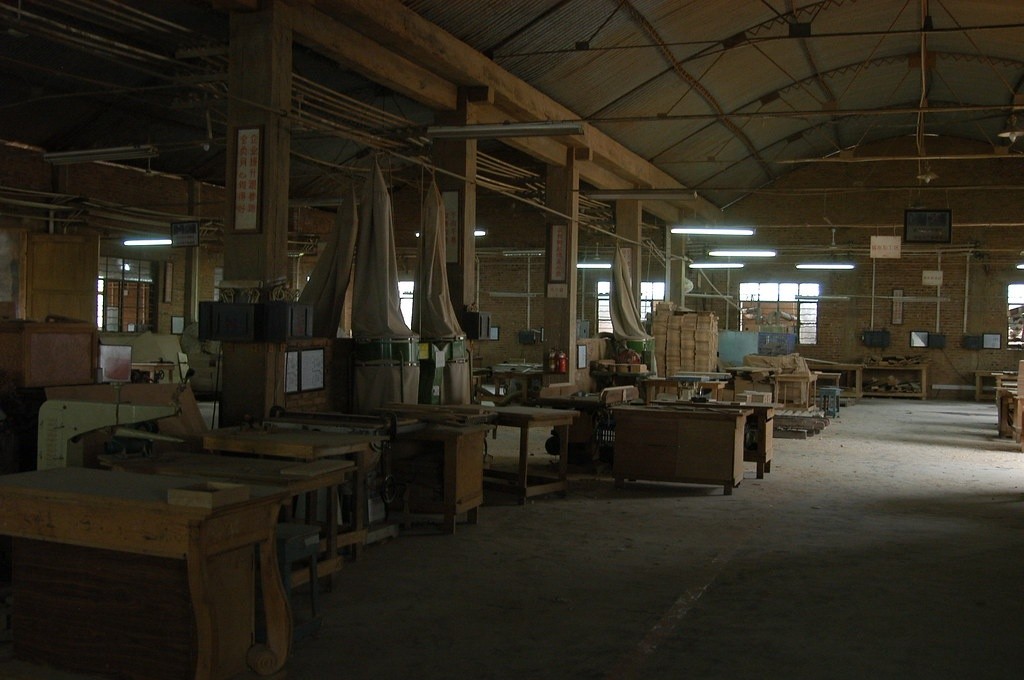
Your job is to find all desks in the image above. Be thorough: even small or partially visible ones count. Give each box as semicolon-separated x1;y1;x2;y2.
0;396;774;679
591;367;842;410
493;371;569;439
131;362;176;384
971;369;1018;402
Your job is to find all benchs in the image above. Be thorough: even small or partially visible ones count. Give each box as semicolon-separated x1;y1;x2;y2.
998;360;1024;443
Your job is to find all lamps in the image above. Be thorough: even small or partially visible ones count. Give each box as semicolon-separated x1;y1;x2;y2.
43;144;159;166
426;122;585;138
916;161;938;182
584;192;698;199
997;114;1024;142
669;227;757;235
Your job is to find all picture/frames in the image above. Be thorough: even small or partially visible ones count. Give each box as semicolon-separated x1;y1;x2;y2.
170;222;199;248
904;209;952;244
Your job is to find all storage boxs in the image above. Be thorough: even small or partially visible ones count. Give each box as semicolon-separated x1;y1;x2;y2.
540;382;572;397
722;376;801;402
591;359;647;373
168;481;249;508
650;302;718;378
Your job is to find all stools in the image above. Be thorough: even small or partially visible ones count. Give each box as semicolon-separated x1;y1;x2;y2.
818;387;841;419
744;391;772;404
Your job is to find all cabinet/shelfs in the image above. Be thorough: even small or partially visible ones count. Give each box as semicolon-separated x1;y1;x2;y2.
805;363;933;403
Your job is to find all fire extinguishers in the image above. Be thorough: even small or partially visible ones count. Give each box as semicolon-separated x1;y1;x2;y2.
559;350;569;374
548;350;557;374
